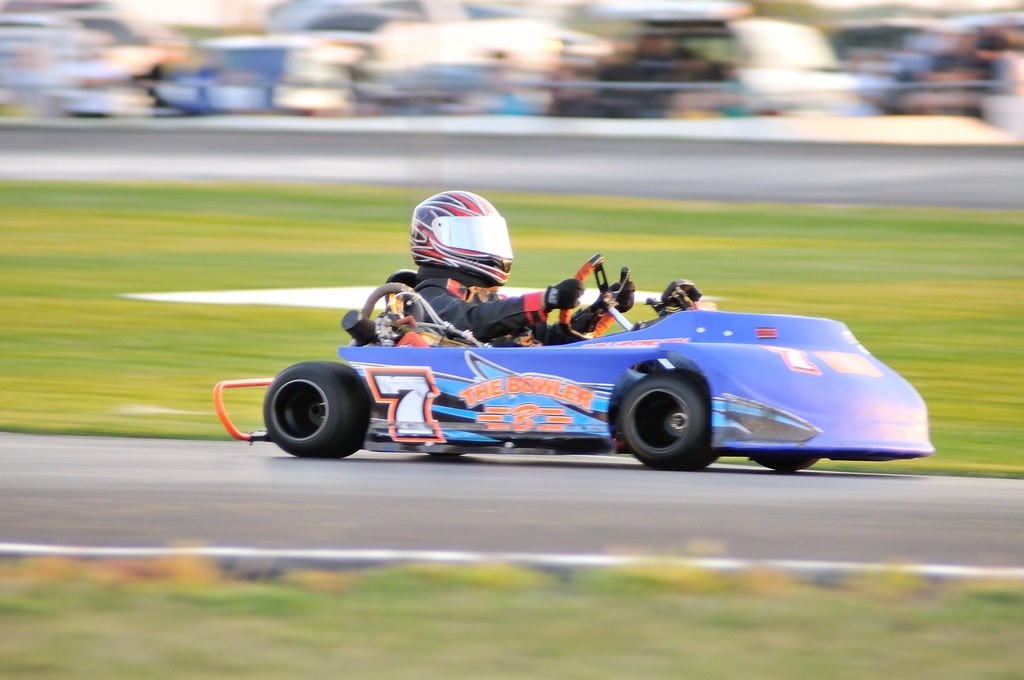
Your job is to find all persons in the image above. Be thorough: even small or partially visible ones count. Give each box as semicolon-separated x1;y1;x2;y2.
403;190;635;347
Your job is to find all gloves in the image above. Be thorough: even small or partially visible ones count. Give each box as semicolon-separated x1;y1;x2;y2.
596;283;636;313
545;278;585;312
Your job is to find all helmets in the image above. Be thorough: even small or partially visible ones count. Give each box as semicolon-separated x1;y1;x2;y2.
410;190;513;289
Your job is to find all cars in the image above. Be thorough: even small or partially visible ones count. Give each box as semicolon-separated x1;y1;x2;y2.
1;0;1024;117
